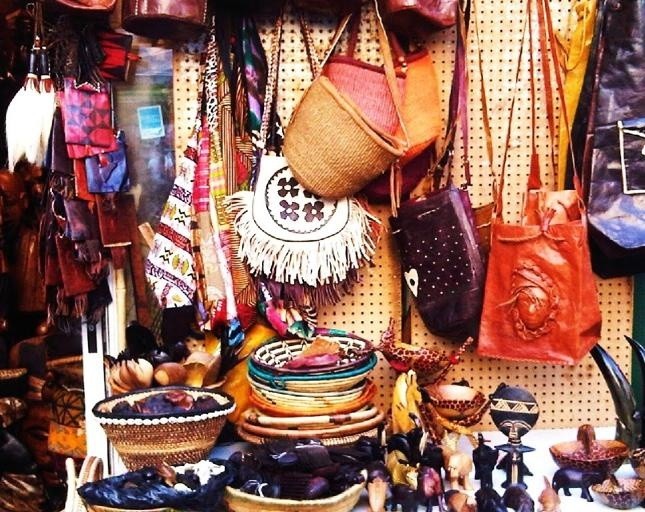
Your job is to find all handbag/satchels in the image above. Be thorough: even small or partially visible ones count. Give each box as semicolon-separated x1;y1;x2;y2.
390;182;602;366
0;0;150;326
150;0;457;346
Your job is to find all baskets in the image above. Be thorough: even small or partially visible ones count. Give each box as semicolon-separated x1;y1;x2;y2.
223;455;369;512
237;328;386;451
78;455;187;511
92;387;237;468
